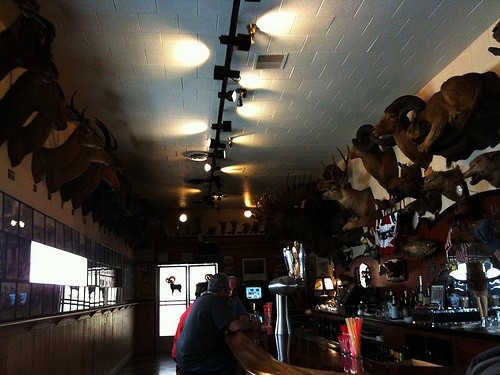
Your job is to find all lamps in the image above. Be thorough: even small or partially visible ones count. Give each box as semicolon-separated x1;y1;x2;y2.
228;137;232;149
208;139;226;149
204;163;220;172
237;89;247;106
218;33;251;51
247;24;257;45
218;91;237;103
211;121;231;132
213;65;240;80
206;150;226;159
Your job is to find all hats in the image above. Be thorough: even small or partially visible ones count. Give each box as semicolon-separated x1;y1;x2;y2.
209;273;230;291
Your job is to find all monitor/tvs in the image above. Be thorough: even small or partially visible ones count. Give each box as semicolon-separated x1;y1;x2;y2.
241;257;268;281
243;285;264;301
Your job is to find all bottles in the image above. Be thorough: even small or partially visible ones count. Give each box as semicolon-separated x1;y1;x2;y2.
312;322;320;336
448;307;480;327
303;328;313;337
388;290;398;320
320;316;328;338
390;344;399;362
384;344;391;363
432;307;447;327
409;351;416;359
358;301;364;316
399;344;409;362
296;326;303;336
410;287;430;307
411;309;431;314
398;291;408;319
364;305;367;316
328;315;341;341
375;342;383;362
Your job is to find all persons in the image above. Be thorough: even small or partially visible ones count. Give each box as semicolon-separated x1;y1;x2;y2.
171;272;260;375
339;275;365;316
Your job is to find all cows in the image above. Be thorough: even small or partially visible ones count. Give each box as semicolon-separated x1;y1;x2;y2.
347;125;400;192
369;92;432;170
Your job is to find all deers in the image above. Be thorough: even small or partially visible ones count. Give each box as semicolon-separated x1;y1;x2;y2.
70;132;122;211
30;88;106;185
45;119;114;194
6;81;68;168
0;67;55;148
0;0;61;85
317;143;376;218
